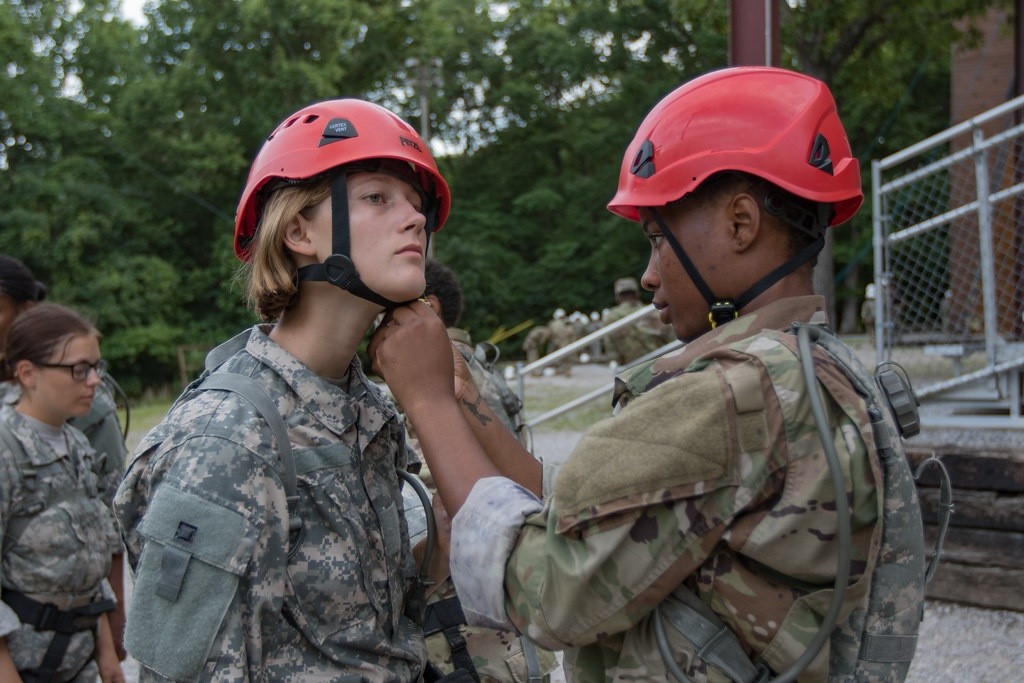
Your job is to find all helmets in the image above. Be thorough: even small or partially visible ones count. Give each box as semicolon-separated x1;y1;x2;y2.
234;98;451;264
606;67;862;228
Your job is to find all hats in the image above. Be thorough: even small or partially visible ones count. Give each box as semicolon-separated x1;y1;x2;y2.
613;277;638;295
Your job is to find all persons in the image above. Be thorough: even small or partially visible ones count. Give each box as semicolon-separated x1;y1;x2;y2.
0;255;129;482
370;260;527;493
112;98;455;677
606;277;676;366
1;301;129;683
522;305;621;377
374;66;954;682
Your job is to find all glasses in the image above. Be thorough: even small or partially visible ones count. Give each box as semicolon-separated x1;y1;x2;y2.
33;360;109;381
